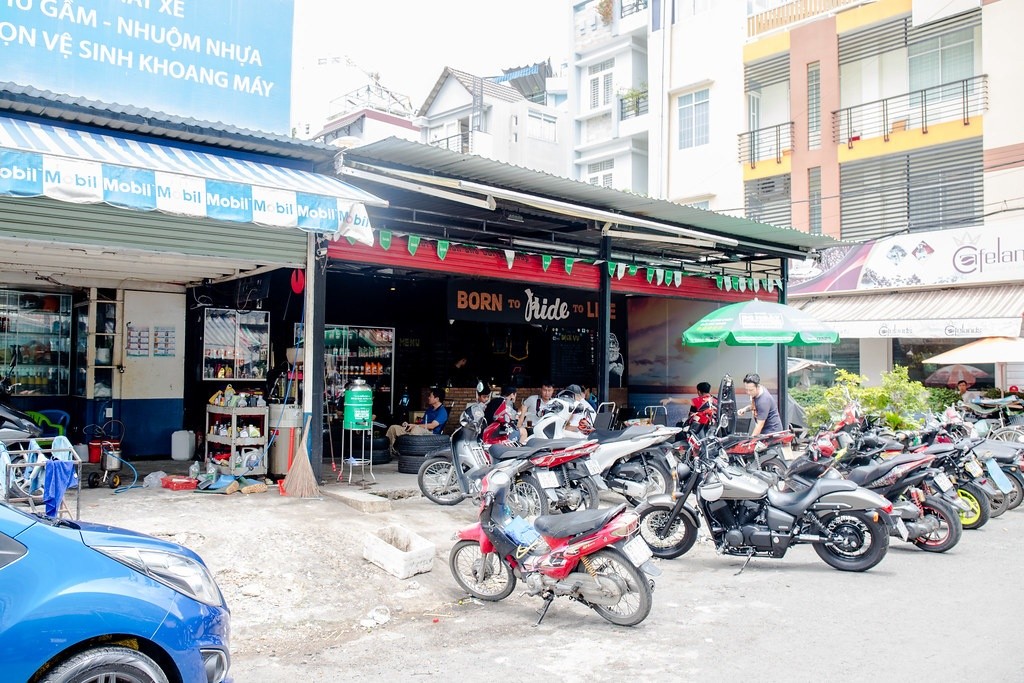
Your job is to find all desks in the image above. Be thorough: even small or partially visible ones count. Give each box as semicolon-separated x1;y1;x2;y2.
984;404;1024;409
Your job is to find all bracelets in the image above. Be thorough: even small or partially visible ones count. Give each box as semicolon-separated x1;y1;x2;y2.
668;397;672;402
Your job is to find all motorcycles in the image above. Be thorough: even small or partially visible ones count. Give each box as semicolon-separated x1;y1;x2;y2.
637;431;890;571
729;386;1023;552
449;464;653;628
419;396;684;508
0;405;52;502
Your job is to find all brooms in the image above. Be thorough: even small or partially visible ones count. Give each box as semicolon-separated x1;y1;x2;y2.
280;414;326;499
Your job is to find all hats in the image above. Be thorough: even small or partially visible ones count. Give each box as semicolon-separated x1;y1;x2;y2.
566;384;581;394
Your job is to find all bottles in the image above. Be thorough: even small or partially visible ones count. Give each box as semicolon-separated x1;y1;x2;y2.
188;461;200;478
0;365;85;394
209;384;266;407
338;362;384;375
98;344;110;363
206;463;216;483
204;362;267;378
210;452;213;462
325;327;358;340
0;311;89;333
324;344;390;358
210;421;260;438
235;447;264;467
1;334;87;352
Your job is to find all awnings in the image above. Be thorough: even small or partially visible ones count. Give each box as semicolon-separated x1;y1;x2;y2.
0;110;389;247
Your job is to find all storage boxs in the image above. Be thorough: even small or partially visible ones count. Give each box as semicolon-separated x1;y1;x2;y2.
409;411;426;424
161;476;198;491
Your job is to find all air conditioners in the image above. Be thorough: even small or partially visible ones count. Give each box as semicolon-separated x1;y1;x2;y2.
758;175;788;200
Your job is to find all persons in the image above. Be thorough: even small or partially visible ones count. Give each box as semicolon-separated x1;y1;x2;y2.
957;381;976;404
386;388;448;454
518;383;555;427
462;384;527;444
660;382;718;431
450;354;467;387
337;382;351;420
737;373;783;436
582;382;597;411
372;392;392;426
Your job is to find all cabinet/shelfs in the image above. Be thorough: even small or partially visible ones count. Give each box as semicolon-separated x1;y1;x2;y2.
293;323;395;426
0;290;116;401
202;308;270;381
205;404;269;476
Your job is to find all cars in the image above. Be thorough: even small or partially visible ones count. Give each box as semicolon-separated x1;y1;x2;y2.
0;504;230;683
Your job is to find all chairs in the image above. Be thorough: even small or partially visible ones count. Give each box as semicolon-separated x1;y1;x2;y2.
25;410;70;446
593;402;620;431
644;405;676;443
734;410;753;437
440;401;455;434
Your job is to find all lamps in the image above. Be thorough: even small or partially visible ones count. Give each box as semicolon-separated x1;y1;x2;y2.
602;222;717;248
390;275;396;290
333;154;497;211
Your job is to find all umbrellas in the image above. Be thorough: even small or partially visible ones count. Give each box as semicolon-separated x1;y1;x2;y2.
926;365;988;389
682;297;840;374
922;337;1024;398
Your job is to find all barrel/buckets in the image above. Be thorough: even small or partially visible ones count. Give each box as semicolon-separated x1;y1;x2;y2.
171;430;195;460
89;439;120;462
74;443;89;463
343;377;373;430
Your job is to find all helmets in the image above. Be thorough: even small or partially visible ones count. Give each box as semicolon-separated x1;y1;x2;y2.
1009;385;1018;392
700;483;724;501
